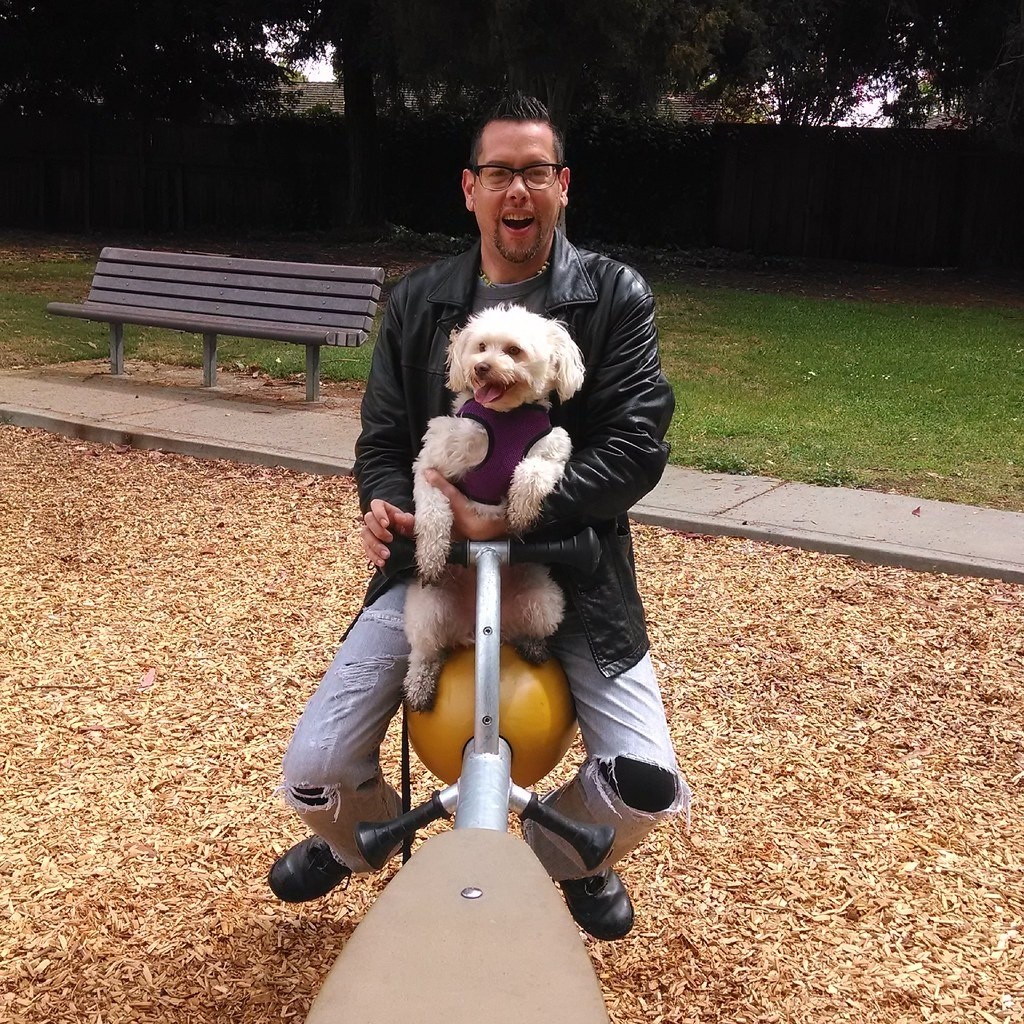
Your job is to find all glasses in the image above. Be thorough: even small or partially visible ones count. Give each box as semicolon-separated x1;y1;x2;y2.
471;163;562;191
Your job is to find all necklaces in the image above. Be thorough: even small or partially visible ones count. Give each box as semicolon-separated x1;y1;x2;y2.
478;258;550;288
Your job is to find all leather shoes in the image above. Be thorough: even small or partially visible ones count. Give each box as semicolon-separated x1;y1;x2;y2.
268;833;353;903
558;865;635;941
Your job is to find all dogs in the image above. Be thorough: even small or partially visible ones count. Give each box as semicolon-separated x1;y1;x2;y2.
402;302;588;713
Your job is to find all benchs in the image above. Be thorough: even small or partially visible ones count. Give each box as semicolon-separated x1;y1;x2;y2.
46;245;387;402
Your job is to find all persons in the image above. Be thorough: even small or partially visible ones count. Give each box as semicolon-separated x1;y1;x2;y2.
269;89;690;941
